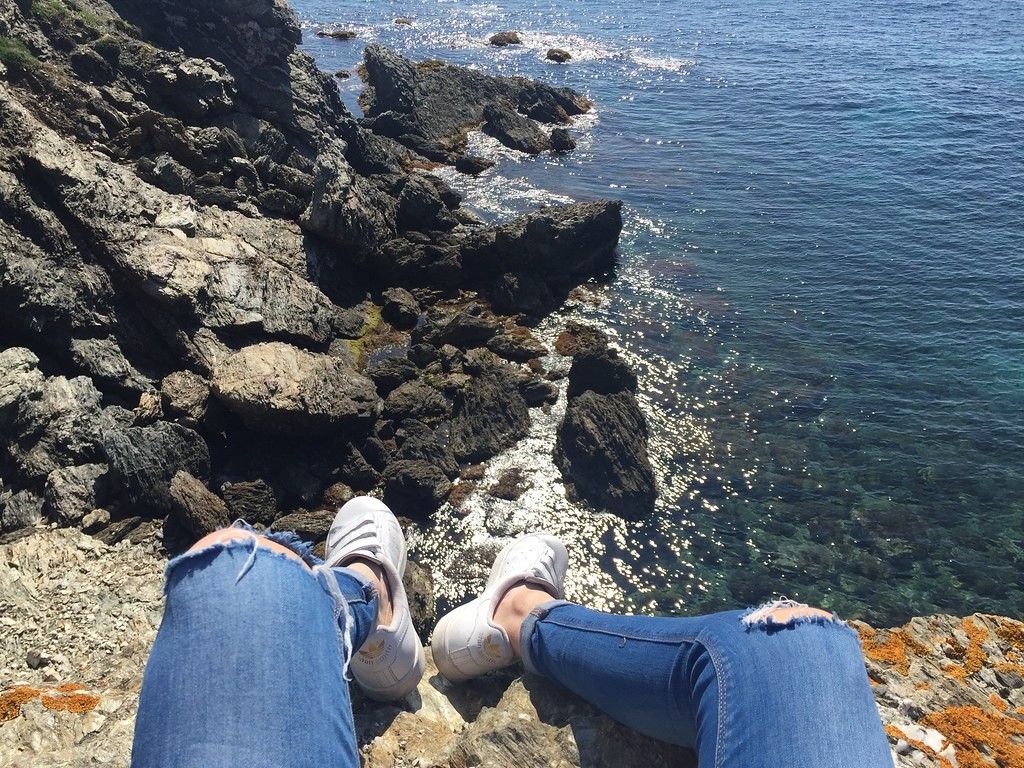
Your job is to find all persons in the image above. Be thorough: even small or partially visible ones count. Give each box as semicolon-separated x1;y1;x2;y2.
129;496;897;768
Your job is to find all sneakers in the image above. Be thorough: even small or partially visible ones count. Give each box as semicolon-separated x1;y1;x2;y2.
324;495;426;704
430;532;569;687
